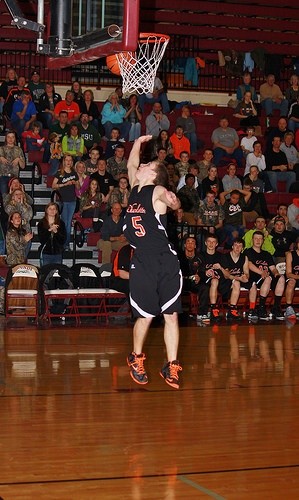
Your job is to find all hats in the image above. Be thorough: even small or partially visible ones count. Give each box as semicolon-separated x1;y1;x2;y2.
159;128;172;137
8;177;17;186
274;216;285;225
49;132;62;140
184;233;198;241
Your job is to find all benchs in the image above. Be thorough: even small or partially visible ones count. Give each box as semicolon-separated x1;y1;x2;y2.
0;100;299;308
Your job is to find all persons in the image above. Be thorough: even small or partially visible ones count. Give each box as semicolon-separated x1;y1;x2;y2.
121;134;183;388
0;68;299;322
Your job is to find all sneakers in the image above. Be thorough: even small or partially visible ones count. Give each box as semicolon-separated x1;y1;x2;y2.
159;360;182;389
127;352;148;385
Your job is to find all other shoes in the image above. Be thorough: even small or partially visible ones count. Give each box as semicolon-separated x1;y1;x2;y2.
228;305;241;320
208;323;219;338
197;314;210;322
270;304;285;320
228;321;240;334
247;309;258;319
284;306;299;320
256;305;269;320
209;304;221;323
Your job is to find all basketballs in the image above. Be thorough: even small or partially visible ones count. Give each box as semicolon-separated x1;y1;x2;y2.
106;51;137;76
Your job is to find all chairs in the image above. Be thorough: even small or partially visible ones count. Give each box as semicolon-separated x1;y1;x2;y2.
3;262;42;327
38;262;80;329
100;262;132;325
72;262;109;327
